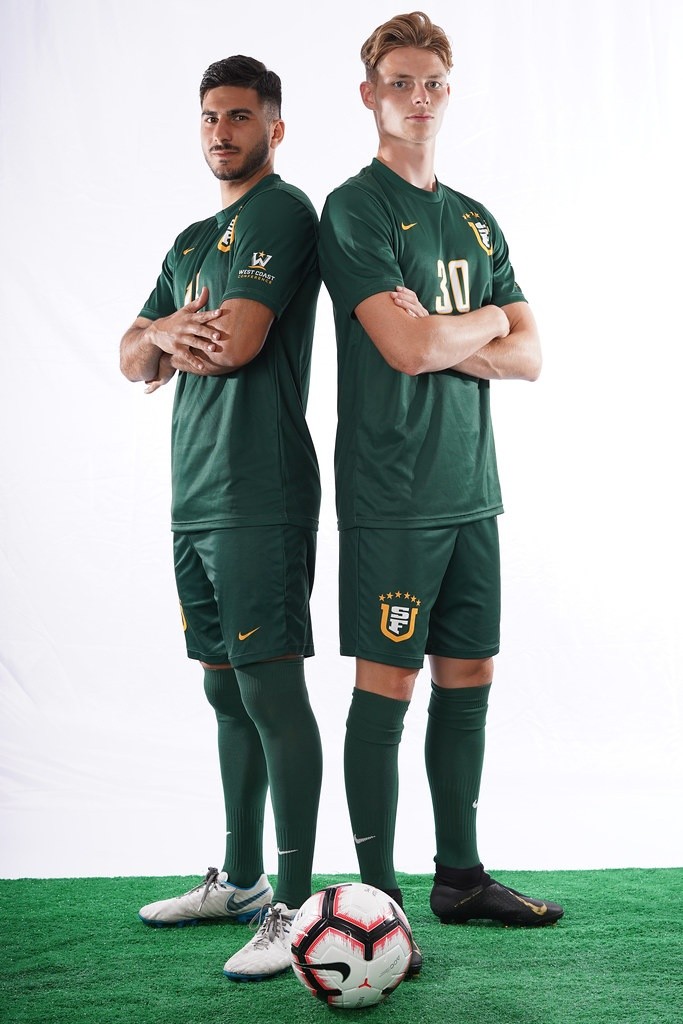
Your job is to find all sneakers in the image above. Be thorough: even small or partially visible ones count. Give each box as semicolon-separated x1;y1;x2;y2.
430;863;564;927
386;888;423;975
222;903;304;981
138;867;274;927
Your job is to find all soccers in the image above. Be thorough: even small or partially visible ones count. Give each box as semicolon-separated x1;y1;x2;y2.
287;881;414;1010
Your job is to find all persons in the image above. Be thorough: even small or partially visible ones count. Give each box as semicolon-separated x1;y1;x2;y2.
120;55;320;982
316;12;563;975
